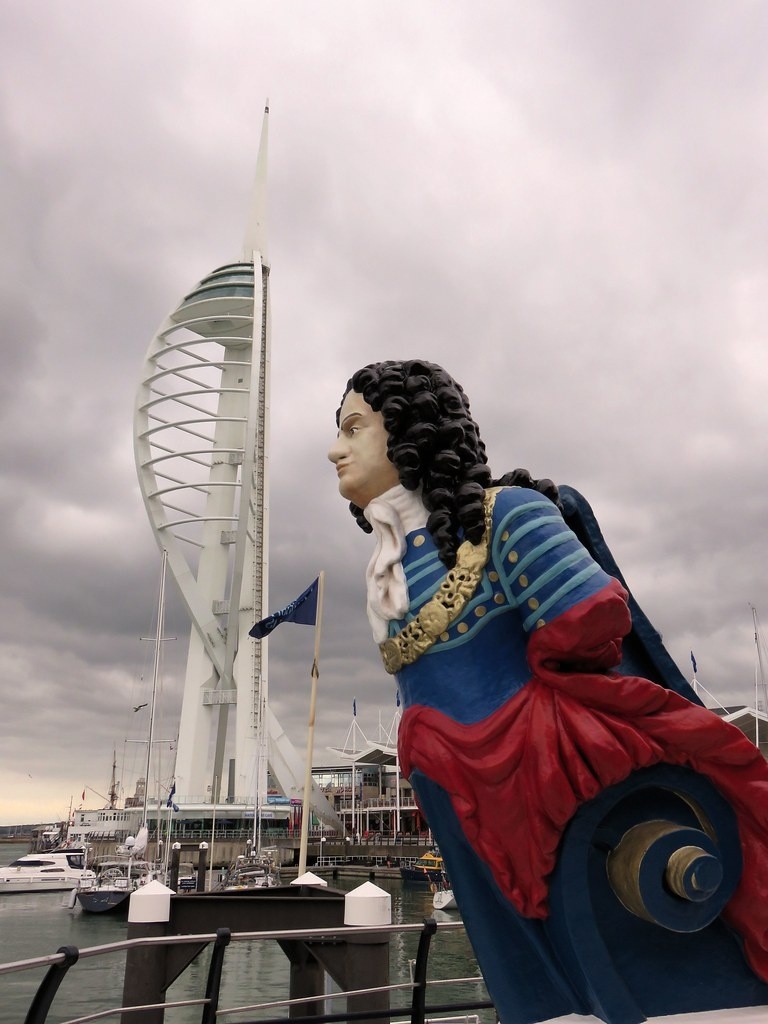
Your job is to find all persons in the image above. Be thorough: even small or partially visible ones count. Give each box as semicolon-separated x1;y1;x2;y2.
327;360;767;1024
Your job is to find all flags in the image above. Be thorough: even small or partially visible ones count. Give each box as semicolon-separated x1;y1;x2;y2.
353;700;357;715
690;653;699;674
82;791;86;799
250;577;318;639
396;690;401;707
166;784;178;812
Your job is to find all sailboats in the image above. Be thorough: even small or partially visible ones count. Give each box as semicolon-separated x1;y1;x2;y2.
223;673;282;892
68;550;178;914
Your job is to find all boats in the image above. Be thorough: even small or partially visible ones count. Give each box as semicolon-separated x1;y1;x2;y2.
399;851;453;881
0;848;96;892
